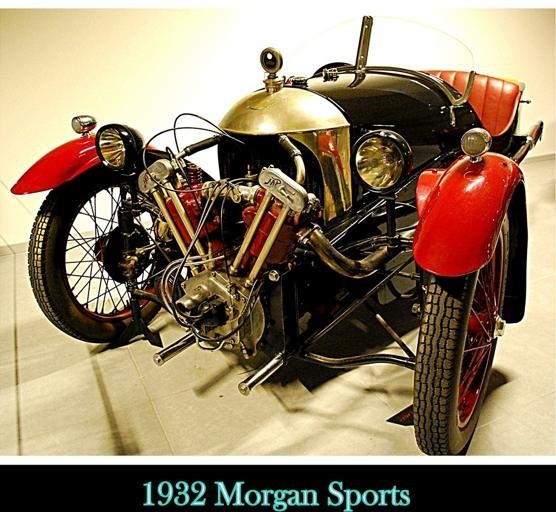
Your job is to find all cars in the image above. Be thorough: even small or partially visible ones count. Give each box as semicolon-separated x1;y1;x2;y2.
7;15;545;455
